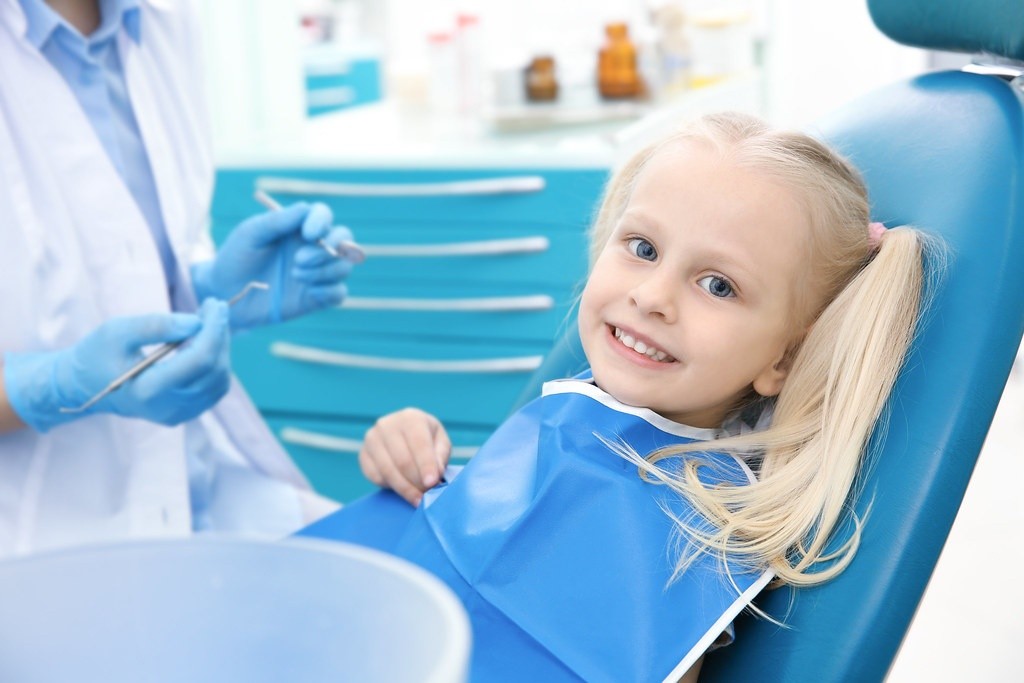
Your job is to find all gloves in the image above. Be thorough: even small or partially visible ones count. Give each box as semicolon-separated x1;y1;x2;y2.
2;298;233;432
188;201;354;328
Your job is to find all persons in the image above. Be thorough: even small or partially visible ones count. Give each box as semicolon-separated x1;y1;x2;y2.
284;110;931;683
0;0;354;540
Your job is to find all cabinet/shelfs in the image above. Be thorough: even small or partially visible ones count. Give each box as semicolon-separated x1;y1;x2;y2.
208;0;932;516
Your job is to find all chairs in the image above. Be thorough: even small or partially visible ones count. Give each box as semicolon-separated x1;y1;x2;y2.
507;0;1024;683
